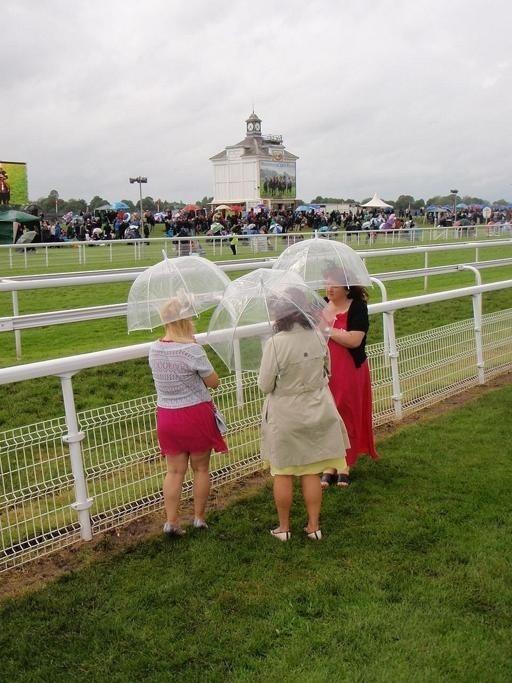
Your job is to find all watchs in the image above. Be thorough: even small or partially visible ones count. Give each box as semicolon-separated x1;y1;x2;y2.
323;325;331;336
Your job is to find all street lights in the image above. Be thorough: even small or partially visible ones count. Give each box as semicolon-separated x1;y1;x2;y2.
449;188;458;220
129;175;148;237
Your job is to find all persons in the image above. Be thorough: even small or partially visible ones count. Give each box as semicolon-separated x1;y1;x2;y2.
13;201;155;254
0;168;11;208
148;301;228;536
317;267;379;490
164;202;511;255
257;289;352;541
262;172;295;197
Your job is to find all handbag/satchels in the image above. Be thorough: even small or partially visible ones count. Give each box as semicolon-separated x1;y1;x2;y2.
213;404;228;434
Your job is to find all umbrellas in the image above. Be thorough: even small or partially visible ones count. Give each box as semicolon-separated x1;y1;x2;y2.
205;266;336;375
125;248;232;336
272;232;373;288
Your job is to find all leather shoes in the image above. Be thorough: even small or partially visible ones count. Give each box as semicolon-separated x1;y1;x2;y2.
337;474;349;486
308;529;321;540
164;524;186;537
193;519;208;528
320;474;334;488
271;530;291;542
